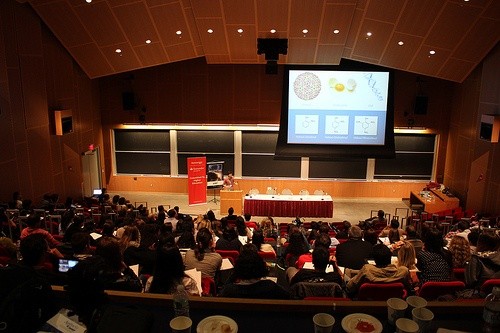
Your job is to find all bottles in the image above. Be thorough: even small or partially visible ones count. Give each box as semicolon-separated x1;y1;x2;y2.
173;284;190;317
482;287;500;333
276;236;281;247
16;240;24;261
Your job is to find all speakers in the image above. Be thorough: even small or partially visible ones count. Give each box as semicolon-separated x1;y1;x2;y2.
121;89;135;109
413;96;428;113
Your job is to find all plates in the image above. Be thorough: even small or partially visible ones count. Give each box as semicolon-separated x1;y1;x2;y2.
341;313;383;333
196;315;238;333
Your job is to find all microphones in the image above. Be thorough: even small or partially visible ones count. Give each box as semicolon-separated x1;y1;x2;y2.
250;189;252;198
300;191;303;200
322;191;324;200
272;189;275;198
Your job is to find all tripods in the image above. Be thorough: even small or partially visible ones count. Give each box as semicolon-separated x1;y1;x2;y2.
209;190;220;205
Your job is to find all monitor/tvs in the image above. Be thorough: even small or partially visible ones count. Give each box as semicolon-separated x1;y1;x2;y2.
57;259;79;274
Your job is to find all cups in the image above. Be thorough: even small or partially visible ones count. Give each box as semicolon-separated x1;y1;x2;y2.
312;313;335;333
170;316;192;333
386;298;408;324
406;296;428;317
394;318;422;333
412;308;434;332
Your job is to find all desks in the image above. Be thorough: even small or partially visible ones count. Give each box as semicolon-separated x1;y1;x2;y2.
244;194;333;218
430;188;459;209
410;190;447;213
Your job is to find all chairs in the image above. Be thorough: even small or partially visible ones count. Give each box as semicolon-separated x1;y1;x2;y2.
299;189;310;195
281;189;293;194
313;189;324;194
0;204;500;298
250;188;260;194
267;187;277;194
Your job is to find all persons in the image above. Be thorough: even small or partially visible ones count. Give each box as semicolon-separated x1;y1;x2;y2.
0;187;500;333
222;171;238;188
